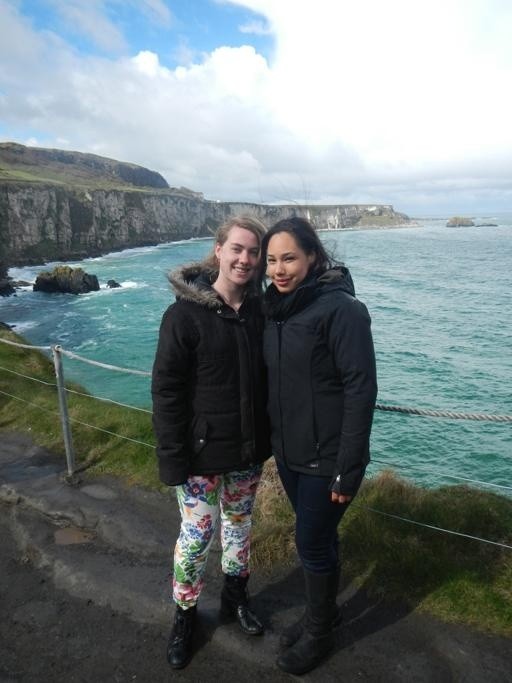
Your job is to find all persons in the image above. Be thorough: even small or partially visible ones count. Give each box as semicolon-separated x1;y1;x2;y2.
151;212;267;671
260;216;378;677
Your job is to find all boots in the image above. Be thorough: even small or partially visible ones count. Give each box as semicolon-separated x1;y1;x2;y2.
167;604;197;669
220;574;265;635
278;563;343;674
